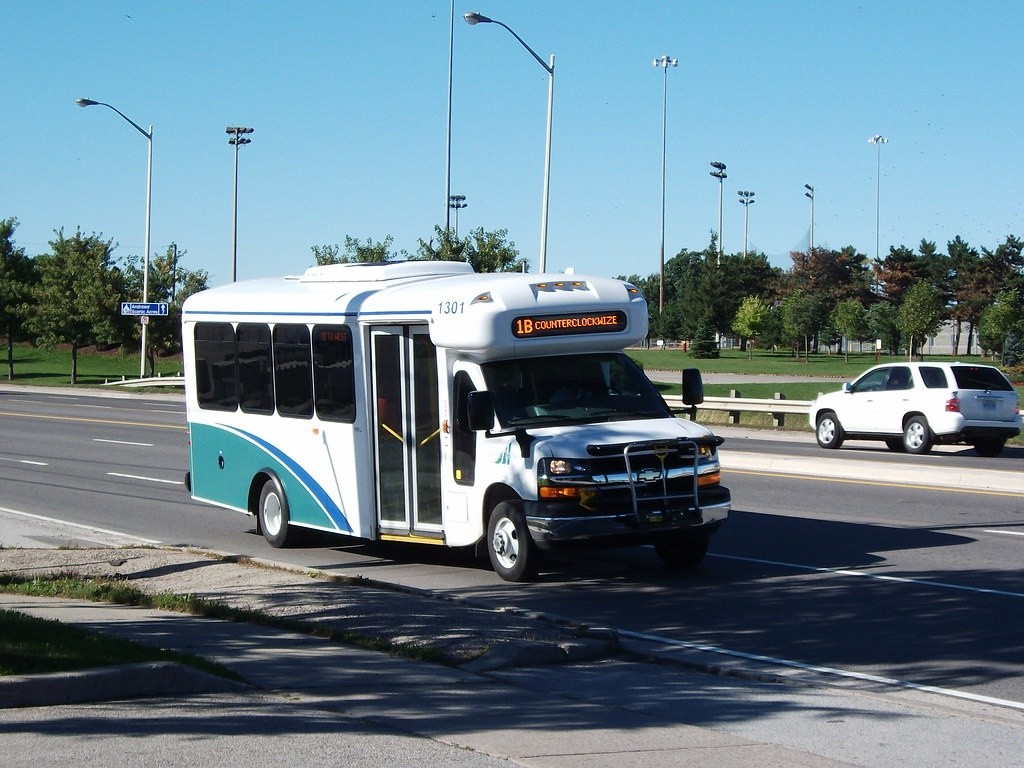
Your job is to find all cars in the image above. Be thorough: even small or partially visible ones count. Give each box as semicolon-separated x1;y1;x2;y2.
809;362;1022;454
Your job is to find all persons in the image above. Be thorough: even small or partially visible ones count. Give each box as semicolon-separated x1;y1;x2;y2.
497;367;544;408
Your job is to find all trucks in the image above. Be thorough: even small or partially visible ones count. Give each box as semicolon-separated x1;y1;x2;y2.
181;257;732;581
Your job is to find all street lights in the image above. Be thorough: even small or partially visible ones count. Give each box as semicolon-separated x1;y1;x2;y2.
225;126;254;284
465;11;555;274
76;99;153;376
804;184;813;253
709;162;727;265
869;135;888;261
653;56;678;316
738;190;755;256
449;194;468;248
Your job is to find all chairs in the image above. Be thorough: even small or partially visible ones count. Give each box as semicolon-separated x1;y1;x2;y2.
542;365;579;405
